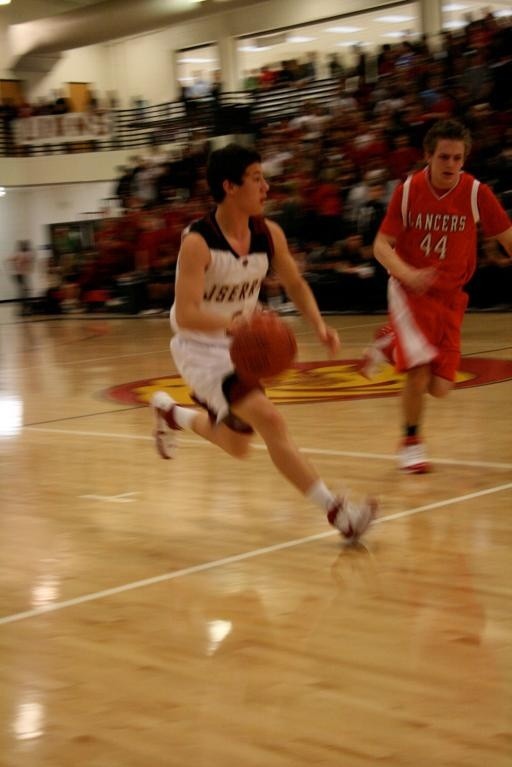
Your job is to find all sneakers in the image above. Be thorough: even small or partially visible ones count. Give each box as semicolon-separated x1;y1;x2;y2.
326;490;379;546
148;389;182;460
392;432;432;475
357;323;395;383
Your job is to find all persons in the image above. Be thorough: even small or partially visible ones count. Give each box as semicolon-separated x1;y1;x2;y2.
150;144;378;543
370;119;512;473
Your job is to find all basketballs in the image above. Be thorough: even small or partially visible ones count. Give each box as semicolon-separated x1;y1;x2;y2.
230;318;297;378
